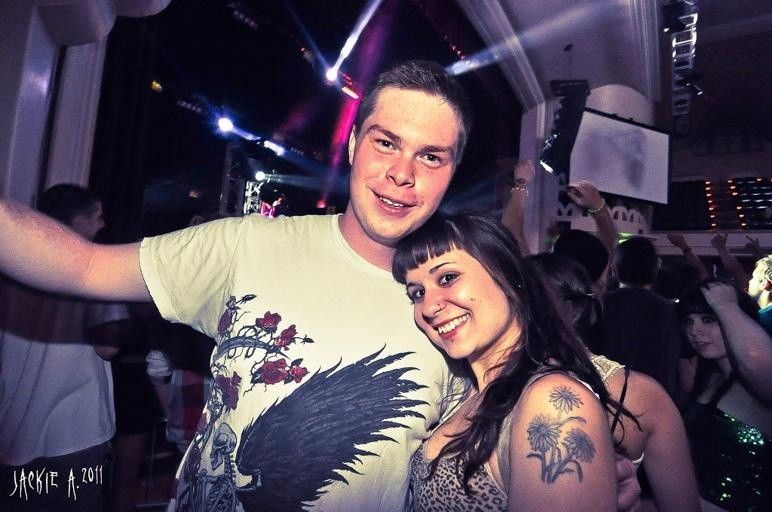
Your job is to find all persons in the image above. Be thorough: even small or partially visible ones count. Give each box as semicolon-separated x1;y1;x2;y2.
1;184;137;512
109;302;175;510
496;155;620;311
143;323;221;453
0;57;645;512
519;231;771;510
391;205;623;512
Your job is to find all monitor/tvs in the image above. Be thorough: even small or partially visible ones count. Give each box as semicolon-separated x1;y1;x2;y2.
567;107;675;207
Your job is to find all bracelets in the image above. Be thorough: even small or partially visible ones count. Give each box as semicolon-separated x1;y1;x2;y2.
512;178;528;187
510;185;530;199
588;198;607;214
